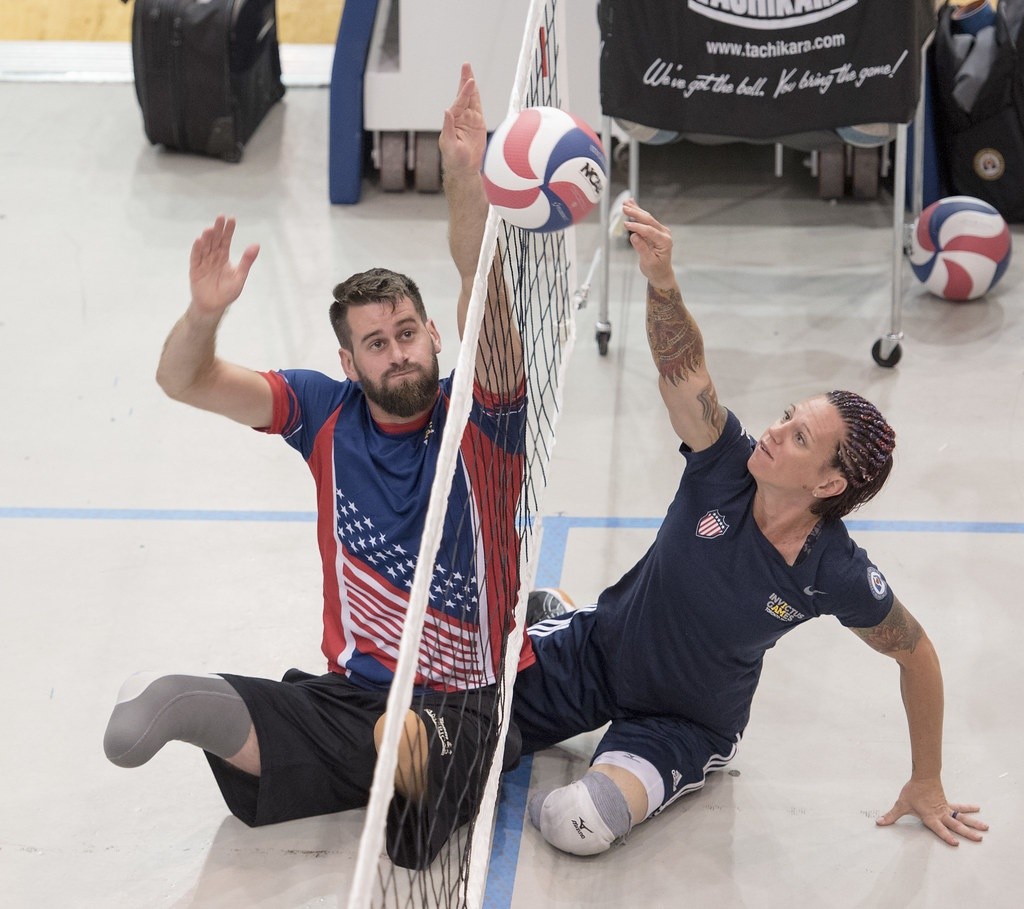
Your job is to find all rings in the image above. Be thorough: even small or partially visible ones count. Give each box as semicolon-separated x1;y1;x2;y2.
952;810;958;819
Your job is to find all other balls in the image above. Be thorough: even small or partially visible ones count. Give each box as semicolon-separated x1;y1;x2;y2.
481;104;611;235
899;196;1016;302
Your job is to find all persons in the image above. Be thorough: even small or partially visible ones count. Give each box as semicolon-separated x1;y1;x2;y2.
512;199;989;859
103;63;525;811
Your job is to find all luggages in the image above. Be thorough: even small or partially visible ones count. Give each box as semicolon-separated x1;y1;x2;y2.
131;0;287;162
